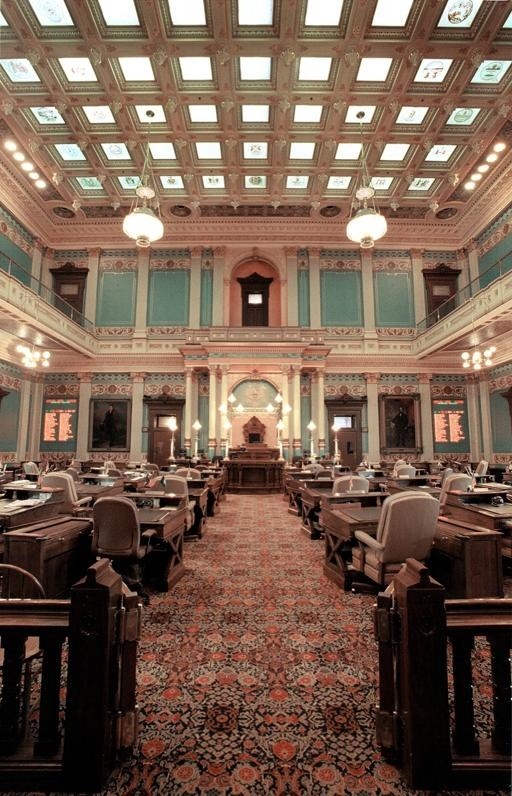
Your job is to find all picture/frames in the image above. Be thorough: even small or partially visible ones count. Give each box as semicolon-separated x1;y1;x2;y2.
86;398;132;453
379;393;423;455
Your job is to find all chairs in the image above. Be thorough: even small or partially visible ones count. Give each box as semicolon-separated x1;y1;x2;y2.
280;458;511;599
0;460;228;600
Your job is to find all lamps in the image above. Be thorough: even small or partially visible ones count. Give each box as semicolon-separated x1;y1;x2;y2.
346;112;388;250
167;420;231;460
276;418;342;463
121;112;166;250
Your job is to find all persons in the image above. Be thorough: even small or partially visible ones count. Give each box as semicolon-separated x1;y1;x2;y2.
100;404;120;447
391;406;408;447
